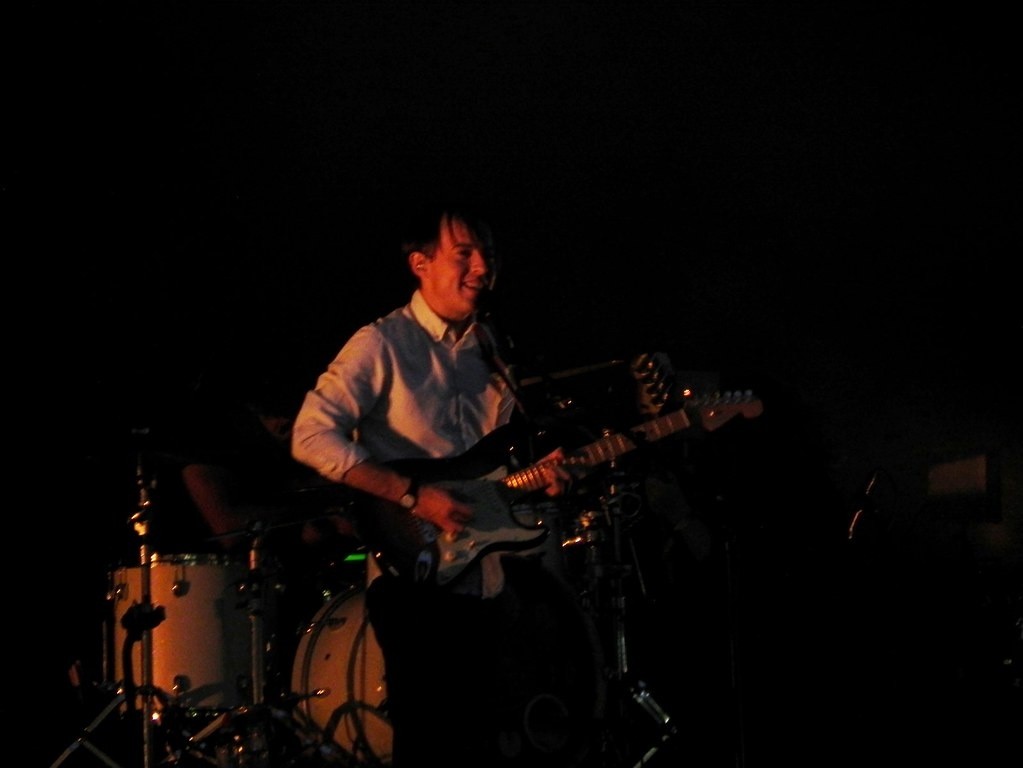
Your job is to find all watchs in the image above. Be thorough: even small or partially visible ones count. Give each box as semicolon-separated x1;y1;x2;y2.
396;480;418;517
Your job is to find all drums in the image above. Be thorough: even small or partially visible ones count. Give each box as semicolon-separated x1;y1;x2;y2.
287;581;396;765
92;537;271;727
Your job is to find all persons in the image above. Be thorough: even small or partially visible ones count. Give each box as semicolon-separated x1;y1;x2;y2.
148;390;359;562
288;196;590;768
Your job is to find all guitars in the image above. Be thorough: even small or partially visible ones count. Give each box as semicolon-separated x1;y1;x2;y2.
343;385;764;598
